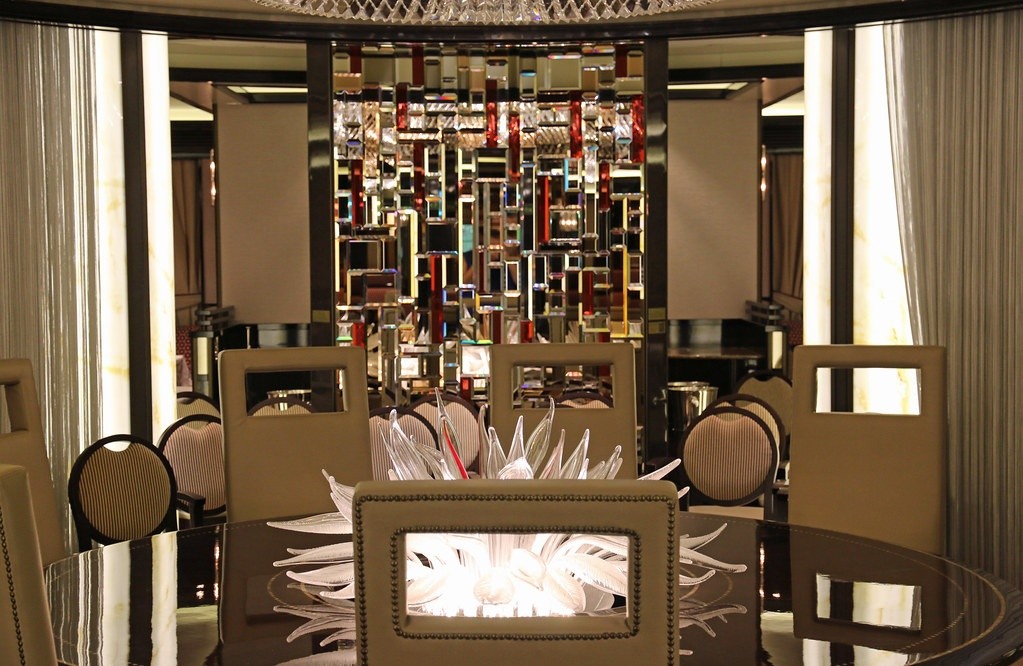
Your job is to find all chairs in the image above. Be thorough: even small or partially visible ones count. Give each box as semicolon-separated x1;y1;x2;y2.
791;520;956;662
216;515;345;644
0;343;951;666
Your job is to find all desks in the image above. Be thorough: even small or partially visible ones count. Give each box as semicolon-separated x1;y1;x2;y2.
209;319;768;516
41;513;1023;666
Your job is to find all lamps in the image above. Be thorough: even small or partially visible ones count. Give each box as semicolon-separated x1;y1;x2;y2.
265;386;748;613
